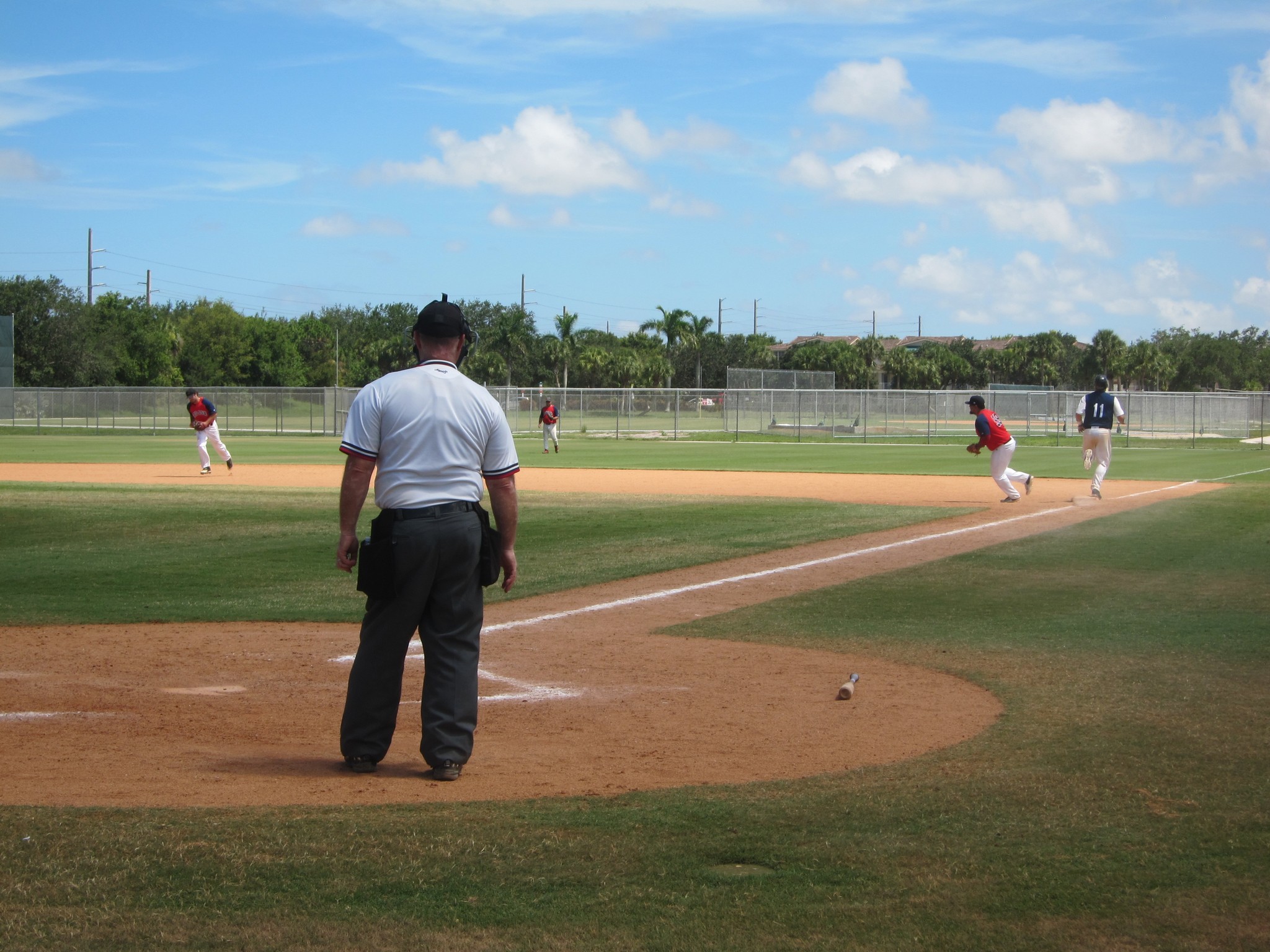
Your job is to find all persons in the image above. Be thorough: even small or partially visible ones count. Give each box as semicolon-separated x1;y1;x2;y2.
538;397;560;454
964;395;1034;503
1075;374;1125;499
337;296;520;781
185;388;233;474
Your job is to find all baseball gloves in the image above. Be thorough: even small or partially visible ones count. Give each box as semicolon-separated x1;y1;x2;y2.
966;444;981;454
547;411;554;420
193;421;206;431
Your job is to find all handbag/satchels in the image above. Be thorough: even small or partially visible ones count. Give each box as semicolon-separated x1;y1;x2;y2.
473;501;501;586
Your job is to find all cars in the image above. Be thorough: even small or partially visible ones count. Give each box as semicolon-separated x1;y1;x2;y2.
687;398;703;406
501;397;529;408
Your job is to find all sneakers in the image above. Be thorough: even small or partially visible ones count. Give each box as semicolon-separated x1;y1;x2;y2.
227;457;233;470
433;762;462;780
1024;474;1034;495
1083;449;1092;470
200;467;211;474
344;755;377;772
1000;496;1020;503
1091;489;1101;500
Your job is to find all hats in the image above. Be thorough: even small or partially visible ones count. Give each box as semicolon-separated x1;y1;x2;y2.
546;397;551;402
186;388;195;398
965;396;984;406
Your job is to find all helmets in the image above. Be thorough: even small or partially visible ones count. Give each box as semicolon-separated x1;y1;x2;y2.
1095;374;1108;386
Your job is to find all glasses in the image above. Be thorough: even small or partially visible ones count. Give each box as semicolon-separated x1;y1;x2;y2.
546;401;550;403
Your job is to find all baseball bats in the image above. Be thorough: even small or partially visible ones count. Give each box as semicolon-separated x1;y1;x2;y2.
839;673;860;700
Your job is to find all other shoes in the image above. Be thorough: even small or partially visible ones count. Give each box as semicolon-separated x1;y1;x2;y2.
555;445;558;453
543;449;548;453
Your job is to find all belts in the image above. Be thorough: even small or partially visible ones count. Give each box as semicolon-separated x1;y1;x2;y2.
1085;426;1107;429
394;501;473;520
207;423;212;426
1003;438;1011;444
546;423;554;425
